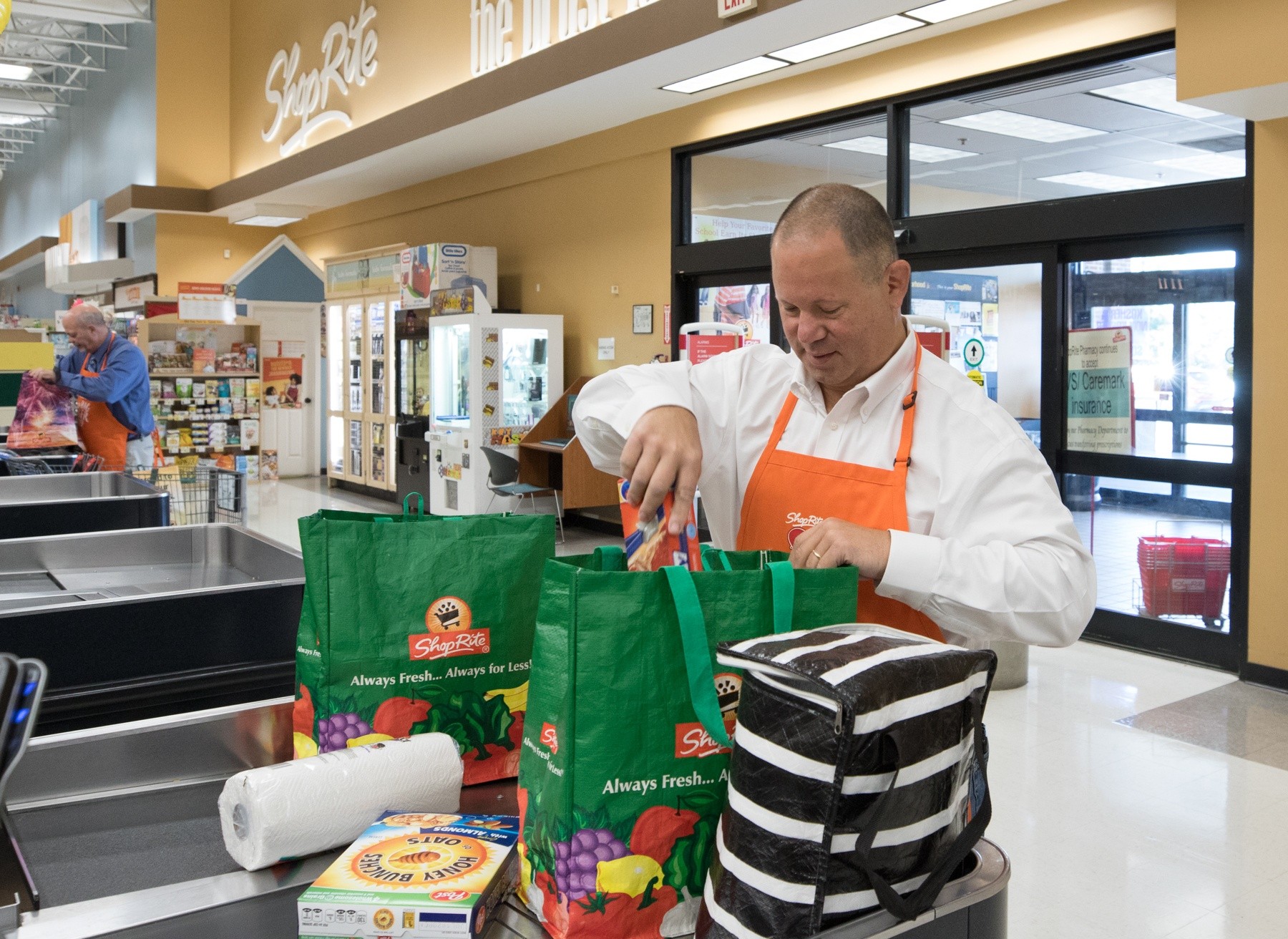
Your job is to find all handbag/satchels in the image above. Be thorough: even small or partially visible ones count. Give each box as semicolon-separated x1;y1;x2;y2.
6;370;86;454
695;621;999;939
518;544;860;939
292;492;557;787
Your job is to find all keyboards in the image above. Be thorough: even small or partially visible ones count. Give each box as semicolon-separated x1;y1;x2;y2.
540;438;572;446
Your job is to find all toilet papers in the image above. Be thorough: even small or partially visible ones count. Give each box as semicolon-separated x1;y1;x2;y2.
218;732;466;873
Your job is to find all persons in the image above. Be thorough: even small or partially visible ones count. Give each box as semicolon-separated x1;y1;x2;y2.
29;303;155;471
415;387;428;413
715;284;770;336
265;373;302;406
570;182;1097;648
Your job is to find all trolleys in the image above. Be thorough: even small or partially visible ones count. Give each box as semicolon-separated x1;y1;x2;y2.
0;448;249;527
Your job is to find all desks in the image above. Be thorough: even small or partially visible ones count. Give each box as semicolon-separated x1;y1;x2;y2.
520;373;620;514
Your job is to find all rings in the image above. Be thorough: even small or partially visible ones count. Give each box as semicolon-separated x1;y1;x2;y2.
812;549;821;559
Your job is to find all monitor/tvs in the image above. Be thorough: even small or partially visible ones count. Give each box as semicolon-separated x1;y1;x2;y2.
567;395;578;431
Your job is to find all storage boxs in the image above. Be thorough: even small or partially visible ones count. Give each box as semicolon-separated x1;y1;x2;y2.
262;449;279;480
296;807;519;939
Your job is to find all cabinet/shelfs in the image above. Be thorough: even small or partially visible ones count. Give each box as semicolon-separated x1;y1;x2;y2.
324;294;400;501
136;319;262;482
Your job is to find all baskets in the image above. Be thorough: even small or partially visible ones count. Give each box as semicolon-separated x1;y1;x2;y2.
1138;536;1232;618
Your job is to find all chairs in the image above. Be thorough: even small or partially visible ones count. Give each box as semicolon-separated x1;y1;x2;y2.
479;446;564;543
